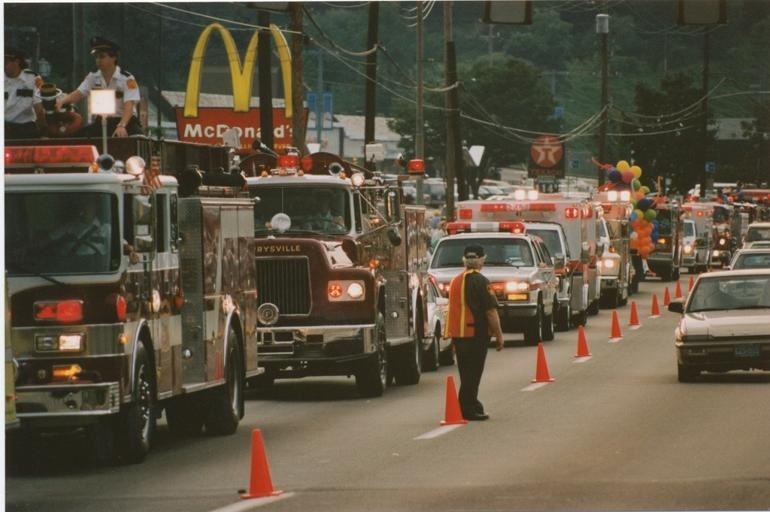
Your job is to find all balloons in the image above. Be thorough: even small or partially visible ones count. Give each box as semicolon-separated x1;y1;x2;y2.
609;161;658;259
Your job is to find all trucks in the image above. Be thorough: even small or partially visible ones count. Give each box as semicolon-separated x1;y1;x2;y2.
453;194;606;331
586;201;644;308
640;181;770;281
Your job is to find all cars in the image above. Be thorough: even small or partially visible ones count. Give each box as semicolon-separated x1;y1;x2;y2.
666;266;770;382
405;269;458;368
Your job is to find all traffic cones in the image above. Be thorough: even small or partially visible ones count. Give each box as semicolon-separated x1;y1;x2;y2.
439;375;468;426
239;429;283;499
675;281;683;298
664;287;673;307
627;301;642;326
531;342;557;384
572;325;593;357
687;277;696;291
650;293;661;316
608;310;624;339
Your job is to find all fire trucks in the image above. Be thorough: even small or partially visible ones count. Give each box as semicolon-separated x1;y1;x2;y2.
232;137;439;400
5;121;262;470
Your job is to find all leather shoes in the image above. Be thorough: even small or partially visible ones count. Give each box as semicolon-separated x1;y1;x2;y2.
465;413;489;420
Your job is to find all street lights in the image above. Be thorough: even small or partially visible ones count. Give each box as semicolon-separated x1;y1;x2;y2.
590;12;611;191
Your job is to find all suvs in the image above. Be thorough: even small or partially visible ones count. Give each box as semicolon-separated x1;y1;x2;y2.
419;221;558;346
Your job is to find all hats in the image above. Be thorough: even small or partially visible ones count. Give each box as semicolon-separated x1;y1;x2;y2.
88;36;119;56
464;245;484;258
5;48;26;58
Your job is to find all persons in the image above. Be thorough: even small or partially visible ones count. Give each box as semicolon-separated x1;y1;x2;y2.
630;249;647;293
759;197;770;222
717;190;728;204
730;181;745;202
305;192;344;235
4;36;141;273
509;245;534;266
444;244;503;421
